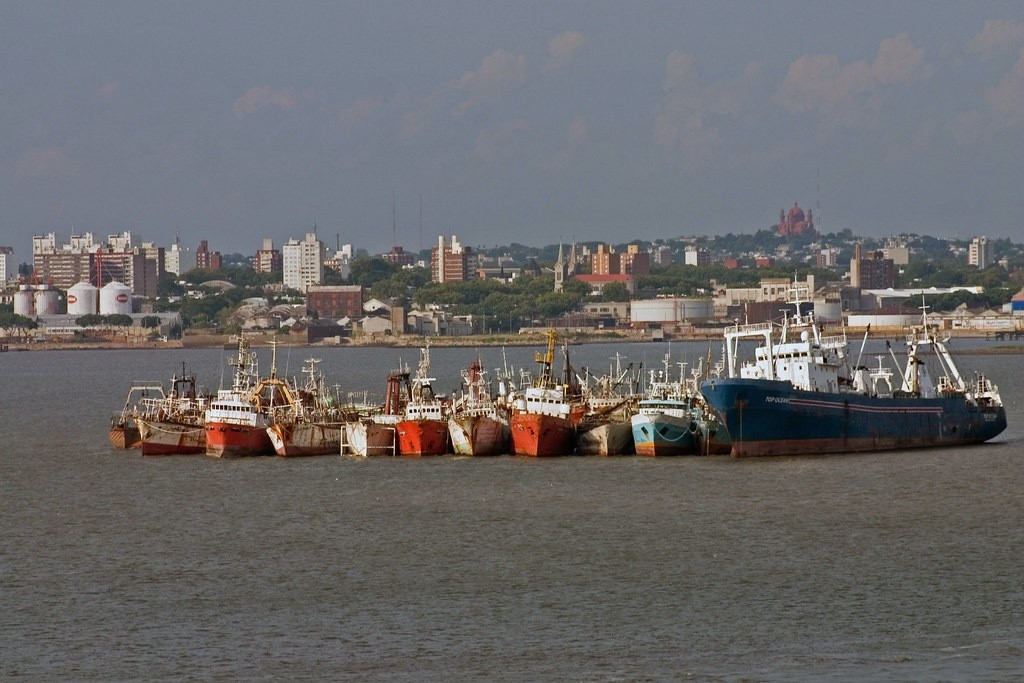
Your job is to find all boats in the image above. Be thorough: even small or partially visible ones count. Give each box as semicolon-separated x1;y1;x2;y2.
696;270;1009;461
107;323;731;460
